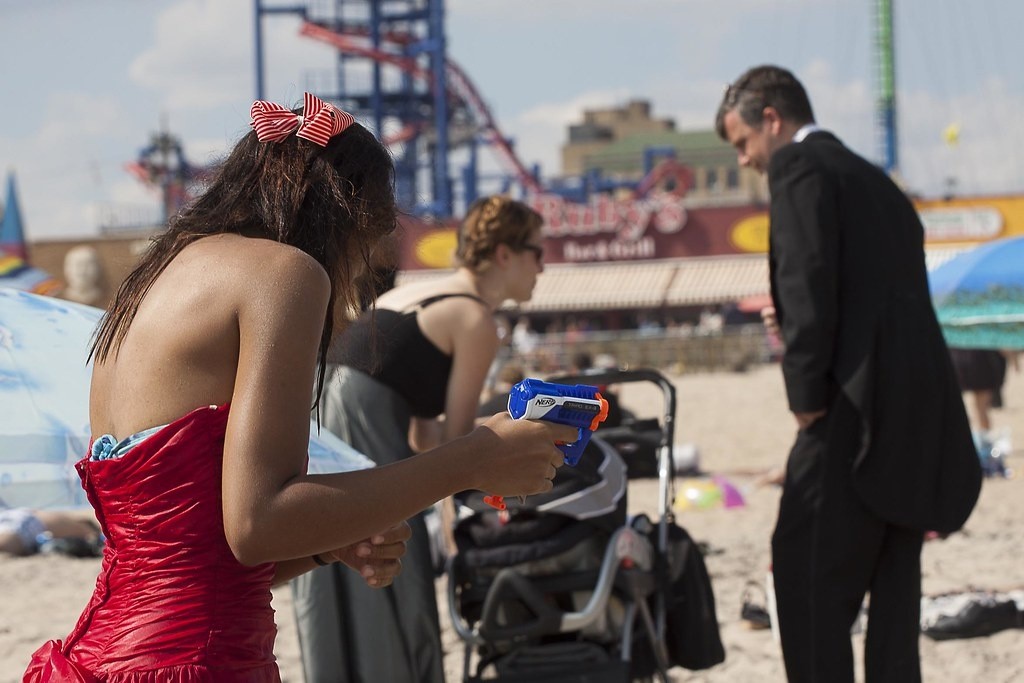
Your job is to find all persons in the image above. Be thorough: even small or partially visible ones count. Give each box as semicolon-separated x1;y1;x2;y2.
949;347;1007;432
22;93;580;683
291;195;543;683
716;67;982;683
0;510;107;561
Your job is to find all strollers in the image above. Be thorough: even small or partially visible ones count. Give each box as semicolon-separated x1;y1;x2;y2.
447;368;678;683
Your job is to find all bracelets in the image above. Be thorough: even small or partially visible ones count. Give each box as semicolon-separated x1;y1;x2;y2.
311;554;329;567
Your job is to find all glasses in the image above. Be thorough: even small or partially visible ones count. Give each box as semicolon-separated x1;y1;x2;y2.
522;244;542;262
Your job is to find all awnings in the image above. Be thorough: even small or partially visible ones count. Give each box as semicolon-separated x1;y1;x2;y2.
367;252;792;313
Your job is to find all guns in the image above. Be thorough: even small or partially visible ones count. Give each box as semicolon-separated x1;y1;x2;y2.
483;379;611;508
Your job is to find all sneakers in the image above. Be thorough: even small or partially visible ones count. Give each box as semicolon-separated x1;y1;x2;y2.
926;599;1022;640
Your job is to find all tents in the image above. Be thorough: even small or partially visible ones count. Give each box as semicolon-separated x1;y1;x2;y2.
0;288;376;509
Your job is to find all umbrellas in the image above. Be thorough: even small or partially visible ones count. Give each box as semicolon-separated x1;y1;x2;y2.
927;233;1024;350
